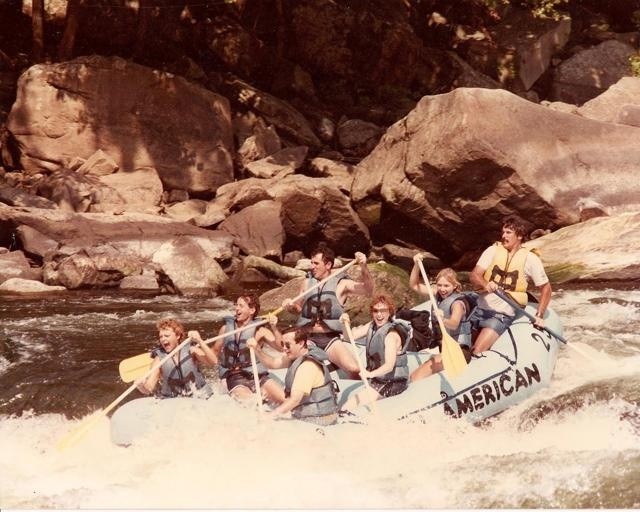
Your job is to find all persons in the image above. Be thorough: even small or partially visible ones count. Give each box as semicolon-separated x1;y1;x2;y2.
402;250;475;385
338;292;415;416
279;240;377;379
129;315;220;401
463;213;553;360
242;325;340;425
207;288;286;407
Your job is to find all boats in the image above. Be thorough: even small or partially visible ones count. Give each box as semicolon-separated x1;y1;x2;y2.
107;290;563;461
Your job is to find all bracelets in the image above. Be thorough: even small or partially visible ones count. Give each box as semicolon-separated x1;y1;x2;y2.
536;311;544;319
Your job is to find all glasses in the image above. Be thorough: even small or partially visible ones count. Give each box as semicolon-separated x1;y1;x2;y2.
374;309;387;313
281;341;290;348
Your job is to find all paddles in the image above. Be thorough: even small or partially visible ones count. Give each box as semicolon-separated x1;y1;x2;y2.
494;288;598;366
50;337;192;467
119;320;270;383
343;319;378;414
417;258;468;389
